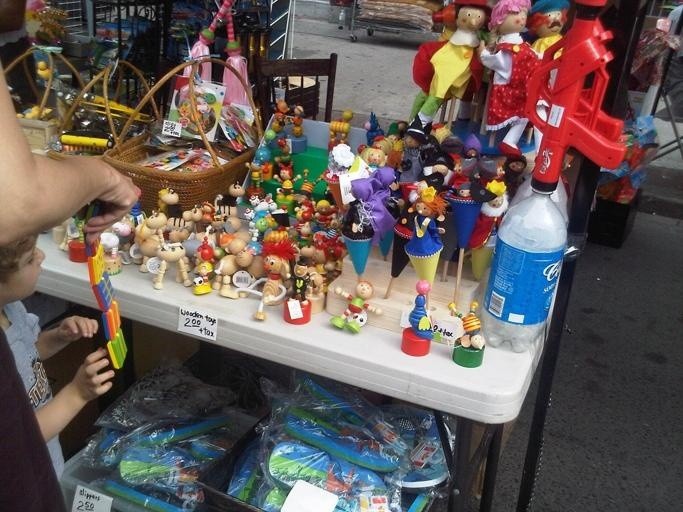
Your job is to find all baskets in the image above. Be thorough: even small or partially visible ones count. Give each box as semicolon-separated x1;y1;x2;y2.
3;46;262;219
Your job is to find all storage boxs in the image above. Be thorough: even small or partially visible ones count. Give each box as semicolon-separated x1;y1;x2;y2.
57;446;150;512
195;412;439;512
587;189;641;249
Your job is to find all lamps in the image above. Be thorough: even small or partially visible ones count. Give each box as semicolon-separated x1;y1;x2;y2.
213;14;269;87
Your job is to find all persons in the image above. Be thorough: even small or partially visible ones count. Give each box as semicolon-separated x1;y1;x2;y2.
330;279;384;334
341;121;525;254
0;51;143;512
406;1;568;152
2;224;115;484
248;160;343;305
191;240;216;296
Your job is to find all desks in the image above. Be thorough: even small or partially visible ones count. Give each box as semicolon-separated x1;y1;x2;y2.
35;232;571;512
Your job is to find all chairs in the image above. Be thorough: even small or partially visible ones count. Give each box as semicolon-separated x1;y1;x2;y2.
252;54;337;131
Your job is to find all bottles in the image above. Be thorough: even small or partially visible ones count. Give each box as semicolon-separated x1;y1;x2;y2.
508;168;569;227
480;177;568;352
